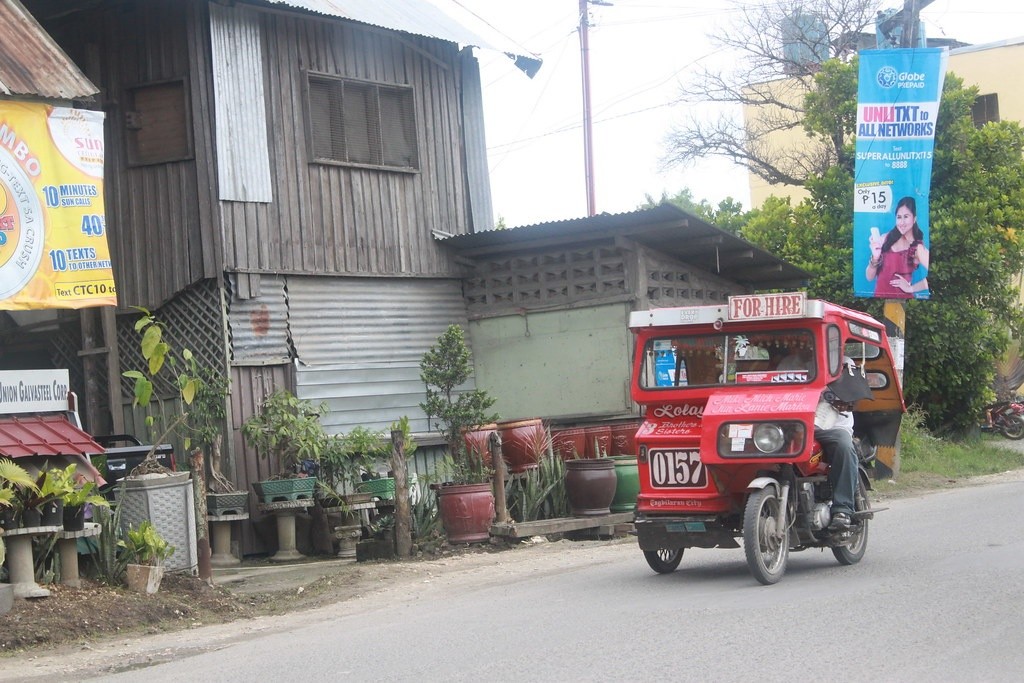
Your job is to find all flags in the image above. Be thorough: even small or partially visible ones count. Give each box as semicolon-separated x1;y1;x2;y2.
853;45;949;303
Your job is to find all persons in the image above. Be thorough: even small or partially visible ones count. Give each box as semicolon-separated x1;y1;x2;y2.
775;330;859;530
865;196;929;299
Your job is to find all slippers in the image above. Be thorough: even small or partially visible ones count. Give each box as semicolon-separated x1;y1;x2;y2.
980;424;993;429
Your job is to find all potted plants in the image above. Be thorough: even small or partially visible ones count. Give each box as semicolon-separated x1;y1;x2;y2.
2;479;20;529
14;472;46;527
191;365;249;516
63;482;108;530
419;324;493;545
40;463;77;525
239;389;329;501
117;520;175;592
342;416;417;499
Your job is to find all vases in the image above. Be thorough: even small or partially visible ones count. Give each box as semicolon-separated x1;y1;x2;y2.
449;423;499;476
498;419;549;471
111;305;201;578
606;454;640;511
611;423;639;455
566;459;618;516
549;428;585;461
584;425;611;460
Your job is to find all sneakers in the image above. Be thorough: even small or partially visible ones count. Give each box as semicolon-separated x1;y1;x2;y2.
828;512;853;531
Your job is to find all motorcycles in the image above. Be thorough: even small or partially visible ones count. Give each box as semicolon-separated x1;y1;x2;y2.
978;398;1024;440
627;291;906;586
